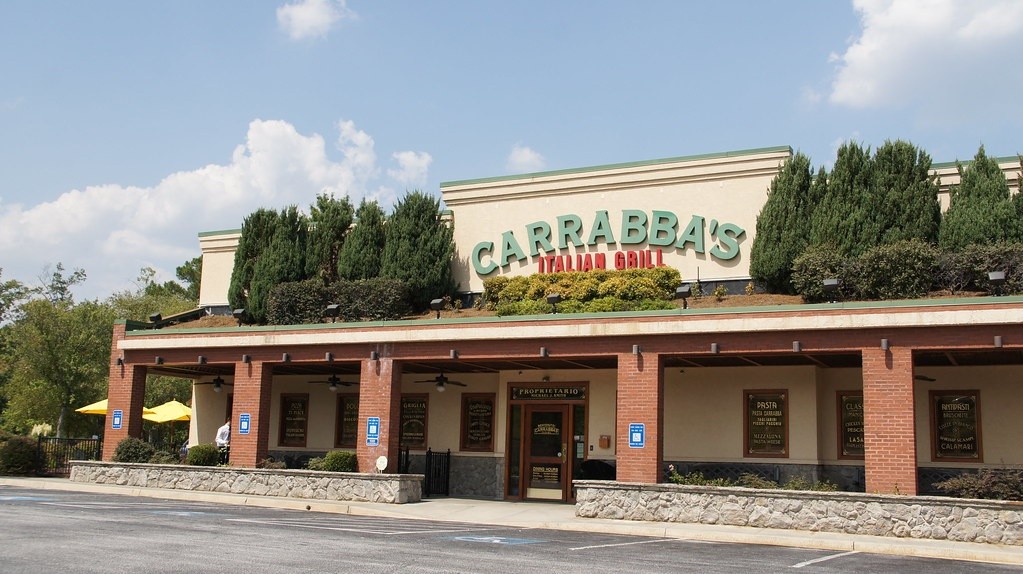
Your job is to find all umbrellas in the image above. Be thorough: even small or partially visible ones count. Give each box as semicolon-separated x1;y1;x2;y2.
75;398;156;415
142;398;192;442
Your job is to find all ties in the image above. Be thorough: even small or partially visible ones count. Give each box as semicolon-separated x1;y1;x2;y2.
228;426;231;439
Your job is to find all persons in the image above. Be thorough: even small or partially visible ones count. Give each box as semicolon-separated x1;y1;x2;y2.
215;419;231;463
179;440;189;464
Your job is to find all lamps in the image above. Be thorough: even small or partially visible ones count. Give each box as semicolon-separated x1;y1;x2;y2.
437;382;446;393
150;313;162;330
823;278;839;303
327;305;340;323
988;271;1005;296
676;287;692;309
328;384;338;392
547;294;561;314
115;335;1002;365
232;309;246;327
213;383;224;393
431;298;445;319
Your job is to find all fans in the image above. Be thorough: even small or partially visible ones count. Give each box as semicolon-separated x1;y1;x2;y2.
413;374;469;388
914;374;936;381
193;375;234;386
308;374;360;386
